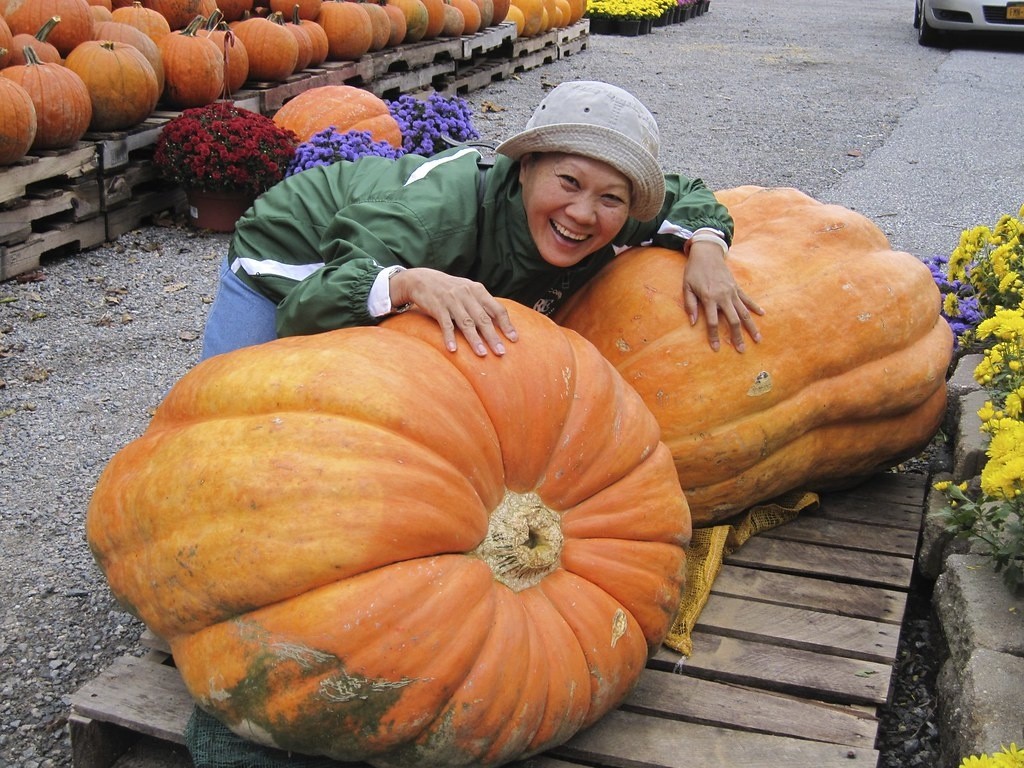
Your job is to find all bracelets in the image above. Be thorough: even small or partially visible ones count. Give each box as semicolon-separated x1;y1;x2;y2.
388;267;415;314
684;234;728;260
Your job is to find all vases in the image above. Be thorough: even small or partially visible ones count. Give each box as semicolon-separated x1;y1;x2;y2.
590;1;710;37
185;187;246;232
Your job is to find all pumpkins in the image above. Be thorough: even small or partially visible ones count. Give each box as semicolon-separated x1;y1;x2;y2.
0;0;587;161
85;295;691;767
556;186;954;521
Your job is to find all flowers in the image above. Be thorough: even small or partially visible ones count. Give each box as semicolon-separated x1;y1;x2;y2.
678;0;698;6
583;0;678;21
922;202;1024;585
152;92;479;192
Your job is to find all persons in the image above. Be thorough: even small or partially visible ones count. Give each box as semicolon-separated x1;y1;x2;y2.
200;78;767;362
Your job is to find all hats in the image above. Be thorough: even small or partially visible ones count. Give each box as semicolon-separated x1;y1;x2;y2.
494;80;666;222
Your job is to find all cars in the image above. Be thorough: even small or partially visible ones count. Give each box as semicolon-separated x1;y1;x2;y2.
913;0;1024;46
